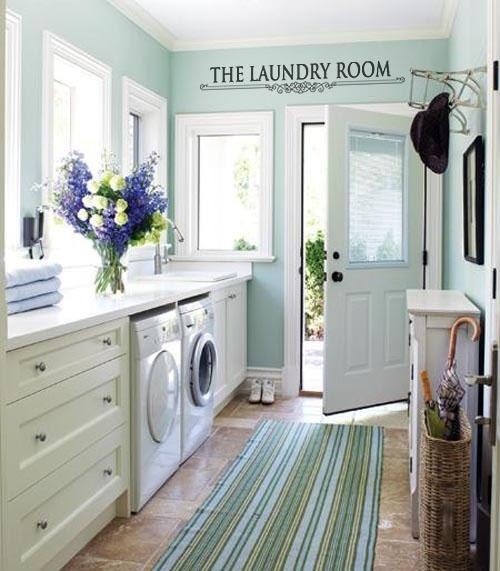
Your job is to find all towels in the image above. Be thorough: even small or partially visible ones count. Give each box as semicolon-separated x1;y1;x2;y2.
4;258;64;316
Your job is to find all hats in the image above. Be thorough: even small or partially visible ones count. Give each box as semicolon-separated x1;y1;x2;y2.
409;91;450;175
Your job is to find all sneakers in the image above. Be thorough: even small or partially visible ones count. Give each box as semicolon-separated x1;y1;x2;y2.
248;379;261;403
261;380;275;405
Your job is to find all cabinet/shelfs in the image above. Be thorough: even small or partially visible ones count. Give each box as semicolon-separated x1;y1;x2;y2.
212;281;247;418
0;316;131;570
406;288;483;544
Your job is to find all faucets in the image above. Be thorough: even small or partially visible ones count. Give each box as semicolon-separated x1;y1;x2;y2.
152;214;184;274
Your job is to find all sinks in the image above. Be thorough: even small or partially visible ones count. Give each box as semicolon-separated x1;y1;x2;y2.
140;271;237;283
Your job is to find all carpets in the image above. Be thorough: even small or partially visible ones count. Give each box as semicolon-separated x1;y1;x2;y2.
150;417;385;570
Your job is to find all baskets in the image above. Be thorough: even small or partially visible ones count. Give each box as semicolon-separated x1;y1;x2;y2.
416;406;473;571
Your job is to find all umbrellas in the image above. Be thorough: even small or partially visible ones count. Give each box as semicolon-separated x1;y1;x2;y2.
421;316;480;439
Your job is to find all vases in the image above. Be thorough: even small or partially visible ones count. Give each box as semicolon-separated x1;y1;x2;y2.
90;239;127;297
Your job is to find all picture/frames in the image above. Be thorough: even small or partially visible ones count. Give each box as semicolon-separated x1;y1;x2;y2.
463;134;484;266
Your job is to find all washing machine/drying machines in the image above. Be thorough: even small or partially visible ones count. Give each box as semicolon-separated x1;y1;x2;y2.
179;295;218;466
130;308;182;515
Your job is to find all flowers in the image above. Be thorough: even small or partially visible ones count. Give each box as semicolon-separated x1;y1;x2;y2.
30;146;168;295
